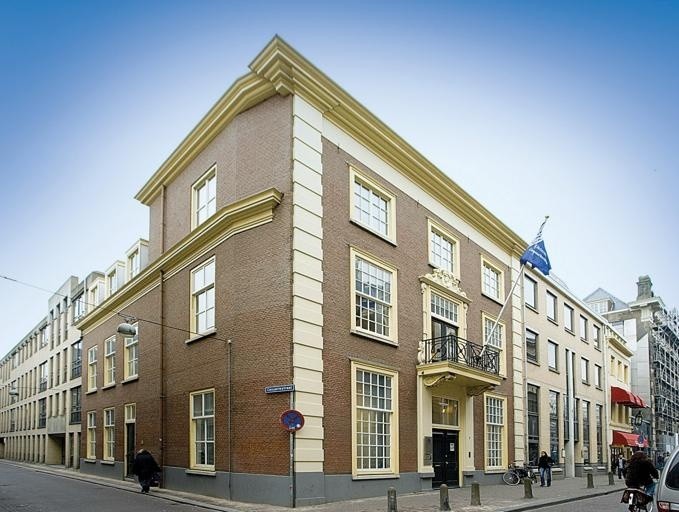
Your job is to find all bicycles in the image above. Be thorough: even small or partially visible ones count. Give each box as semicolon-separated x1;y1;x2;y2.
503;462;537;485
628;477;654;512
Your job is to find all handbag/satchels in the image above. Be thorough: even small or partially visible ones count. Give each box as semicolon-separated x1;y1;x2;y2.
620;488;653;505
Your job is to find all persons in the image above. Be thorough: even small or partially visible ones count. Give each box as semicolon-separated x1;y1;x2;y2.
538;451;556;486
133;447;161;493
624;450;659;509
655;451;671;472
614;452;628;479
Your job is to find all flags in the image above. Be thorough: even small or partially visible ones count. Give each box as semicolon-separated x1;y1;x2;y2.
518;219;552;276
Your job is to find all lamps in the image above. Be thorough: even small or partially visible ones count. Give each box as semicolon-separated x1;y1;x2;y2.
634;408;643;428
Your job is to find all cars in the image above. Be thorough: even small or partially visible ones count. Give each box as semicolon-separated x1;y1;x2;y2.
657;443;679;511
